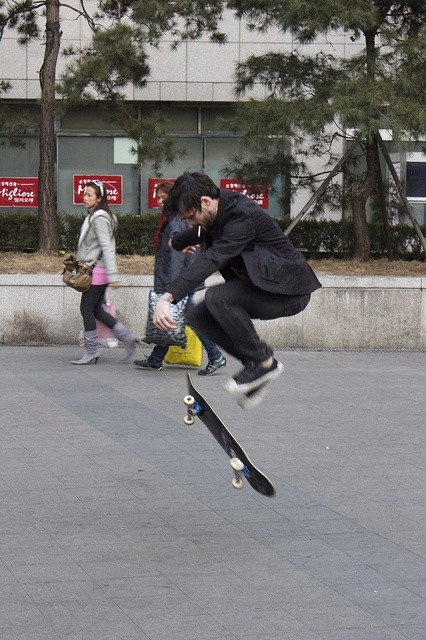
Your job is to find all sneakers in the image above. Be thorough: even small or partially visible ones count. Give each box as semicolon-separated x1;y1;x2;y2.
237;374;279;409
223;358;281;394
133;359;162;371
198;355;226;376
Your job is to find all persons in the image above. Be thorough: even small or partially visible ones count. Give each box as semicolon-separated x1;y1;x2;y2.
65;181;139;365
152;173;322;411
134;181;227;375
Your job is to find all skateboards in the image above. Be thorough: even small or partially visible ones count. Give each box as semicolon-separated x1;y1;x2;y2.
184;371;277;498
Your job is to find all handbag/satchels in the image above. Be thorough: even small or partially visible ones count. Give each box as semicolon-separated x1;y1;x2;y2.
142;289;189;347
60;254;92;292
96;303;118;348
164;325;203;367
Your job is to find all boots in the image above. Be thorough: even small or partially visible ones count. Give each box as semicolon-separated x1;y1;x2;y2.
113;322;142;361
70;329;100;365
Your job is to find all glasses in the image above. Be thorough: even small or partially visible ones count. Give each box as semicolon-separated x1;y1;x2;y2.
181;208;197;223
94;180;104;197
